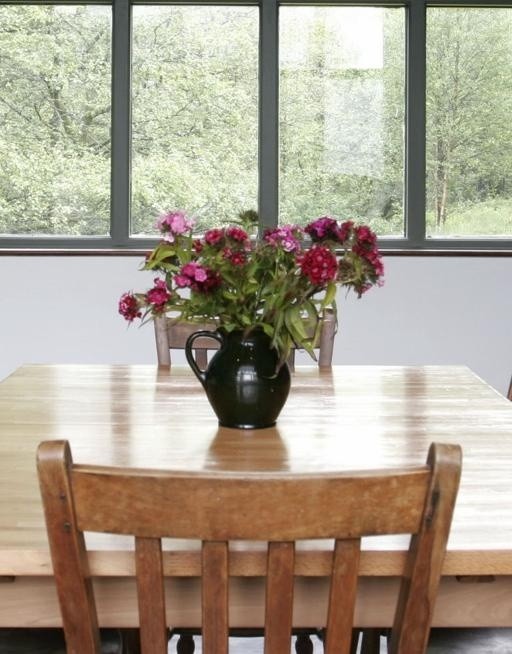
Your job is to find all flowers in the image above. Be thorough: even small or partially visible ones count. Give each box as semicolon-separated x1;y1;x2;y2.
118;210;385;378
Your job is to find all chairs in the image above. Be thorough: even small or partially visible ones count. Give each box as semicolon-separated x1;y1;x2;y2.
154;308;336;368
38;440;462;654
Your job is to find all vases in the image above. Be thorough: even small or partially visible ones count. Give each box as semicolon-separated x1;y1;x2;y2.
185;323;291;429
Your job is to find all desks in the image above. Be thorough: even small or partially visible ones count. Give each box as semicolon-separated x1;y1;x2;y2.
0;363;512;628
